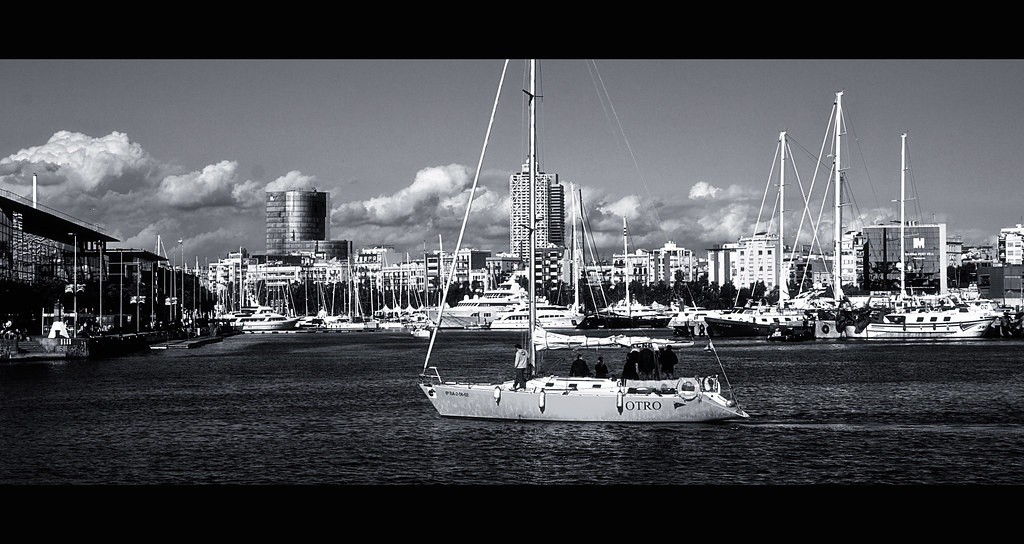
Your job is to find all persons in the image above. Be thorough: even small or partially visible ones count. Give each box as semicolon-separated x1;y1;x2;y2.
595;357;609;378
568;354;590;377
621;344;678;387
918;301;929;313
509;345;530;391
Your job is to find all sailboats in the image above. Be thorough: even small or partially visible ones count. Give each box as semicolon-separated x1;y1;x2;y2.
205;90;1021;342
415;61;750;422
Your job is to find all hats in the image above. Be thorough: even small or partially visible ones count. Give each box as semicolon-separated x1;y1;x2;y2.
658;346;664;349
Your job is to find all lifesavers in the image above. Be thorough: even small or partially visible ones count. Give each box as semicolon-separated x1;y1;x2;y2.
699;325;705;336
821;325;829;334
677;378;700;401
704;376;718;391
739;318;743;322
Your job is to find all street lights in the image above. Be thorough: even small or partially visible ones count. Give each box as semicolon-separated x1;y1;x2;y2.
67;233;77;338
95;242;103;328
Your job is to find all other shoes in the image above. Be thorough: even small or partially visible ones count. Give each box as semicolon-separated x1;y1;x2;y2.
517;387;524;391
509;387;515;390
